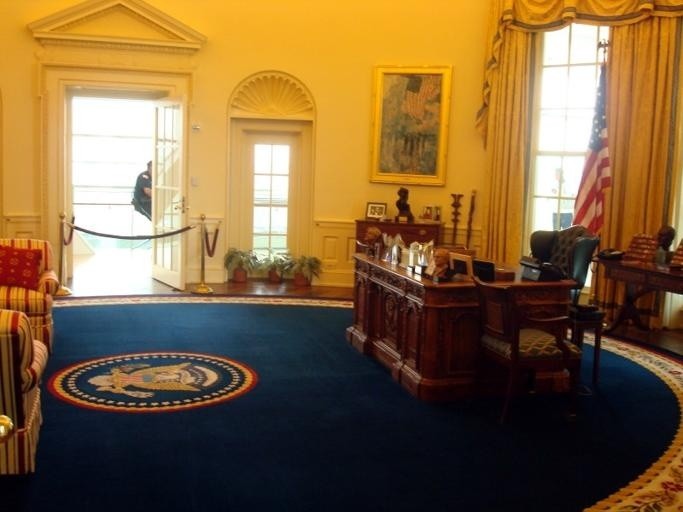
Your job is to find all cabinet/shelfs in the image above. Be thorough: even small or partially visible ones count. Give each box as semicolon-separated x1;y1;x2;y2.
353;219;441;253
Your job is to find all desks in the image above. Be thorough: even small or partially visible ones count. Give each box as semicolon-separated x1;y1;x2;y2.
595;256;683;340
343;240;585;399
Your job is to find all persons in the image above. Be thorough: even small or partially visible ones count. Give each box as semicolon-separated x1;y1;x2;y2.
432;248;449;277
134;161;152;218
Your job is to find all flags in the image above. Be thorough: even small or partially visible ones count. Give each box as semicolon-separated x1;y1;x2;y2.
569;67;613;241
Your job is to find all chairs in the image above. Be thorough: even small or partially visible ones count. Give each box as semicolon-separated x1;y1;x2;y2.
1;235;60;476
471;275;585;425
518;222;600;323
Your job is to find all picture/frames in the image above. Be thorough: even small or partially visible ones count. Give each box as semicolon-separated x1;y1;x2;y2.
367;62;452;186
366;202;387;220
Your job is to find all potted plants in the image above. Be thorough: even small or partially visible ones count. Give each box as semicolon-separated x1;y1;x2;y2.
222;247;323;290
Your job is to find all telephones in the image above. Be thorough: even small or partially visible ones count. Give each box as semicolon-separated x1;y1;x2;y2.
519;256;563;282
598;248;625;260
